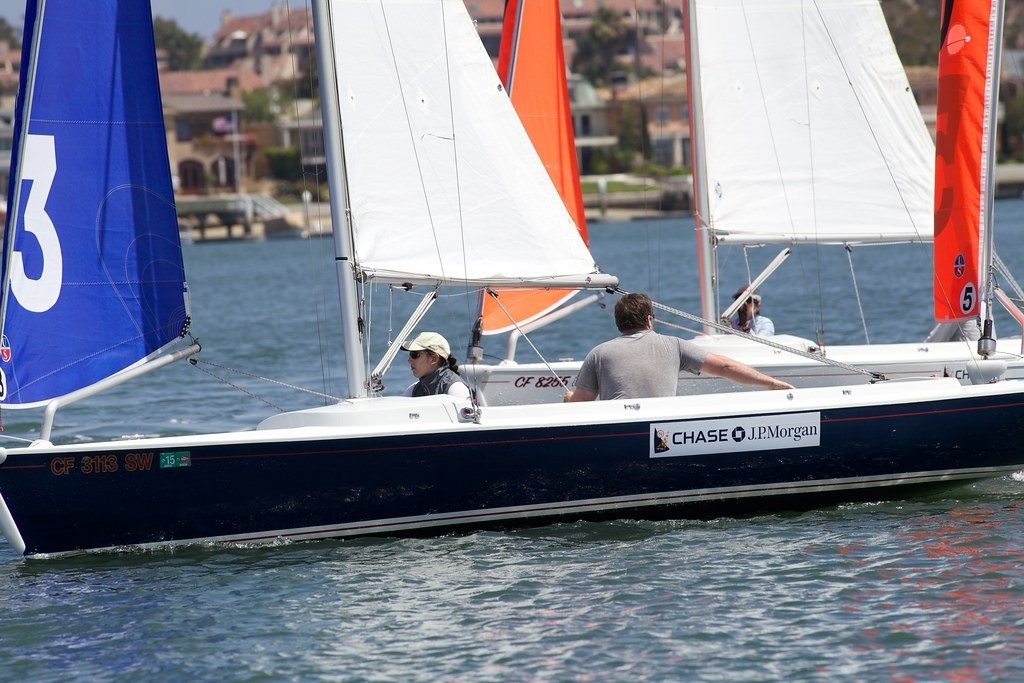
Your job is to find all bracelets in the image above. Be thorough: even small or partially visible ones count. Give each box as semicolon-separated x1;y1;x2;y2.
743;328;748;332
743;322;748;326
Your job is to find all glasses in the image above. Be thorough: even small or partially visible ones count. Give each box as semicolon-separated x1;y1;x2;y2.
409;352;432;359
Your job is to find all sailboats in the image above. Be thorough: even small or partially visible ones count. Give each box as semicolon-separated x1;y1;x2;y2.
2;0;1024;558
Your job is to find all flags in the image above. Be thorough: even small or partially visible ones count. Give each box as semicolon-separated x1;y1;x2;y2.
213;115;232;132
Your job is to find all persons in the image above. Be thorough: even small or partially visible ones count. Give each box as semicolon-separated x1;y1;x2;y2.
564;293;795;402
724;286;774;336
400;332;475;399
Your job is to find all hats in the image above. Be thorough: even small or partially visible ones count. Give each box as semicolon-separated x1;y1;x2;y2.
401;331;451;360
732;286;761;302
